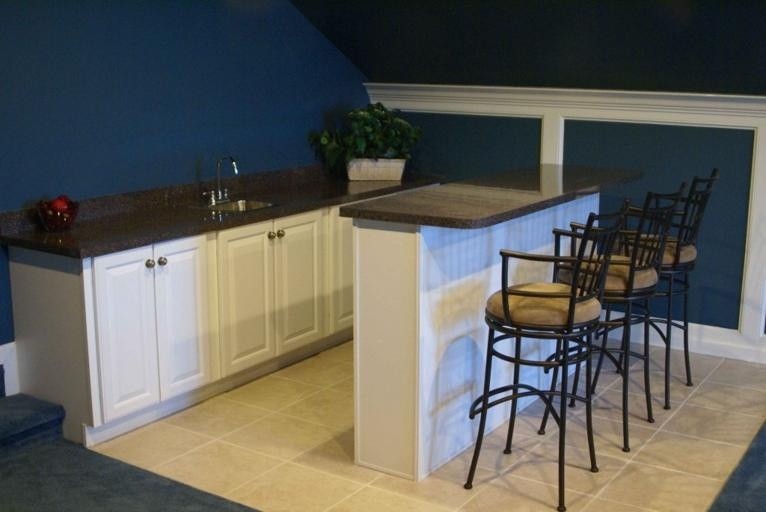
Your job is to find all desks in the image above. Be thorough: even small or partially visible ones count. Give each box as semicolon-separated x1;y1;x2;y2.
334;163;647;483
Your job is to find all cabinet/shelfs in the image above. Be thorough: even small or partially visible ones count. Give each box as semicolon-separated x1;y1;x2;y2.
9;234;209;430
328;206;354;334
216;209;324;381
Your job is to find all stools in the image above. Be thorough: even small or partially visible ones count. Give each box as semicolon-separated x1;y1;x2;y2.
539;182;688;453
592;170;718;411
464;198;630;511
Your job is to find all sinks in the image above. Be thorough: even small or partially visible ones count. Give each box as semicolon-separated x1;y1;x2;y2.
209;198;271;213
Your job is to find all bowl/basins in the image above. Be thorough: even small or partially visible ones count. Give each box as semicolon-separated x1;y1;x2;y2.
37;198;80;233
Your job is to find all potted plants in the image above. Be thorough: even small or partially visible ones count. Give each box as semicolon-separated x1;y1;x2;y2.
310;96;423;183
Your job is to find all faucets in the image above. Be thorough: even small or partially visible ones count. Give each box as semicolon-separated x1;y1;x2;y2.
216;155;239;201
